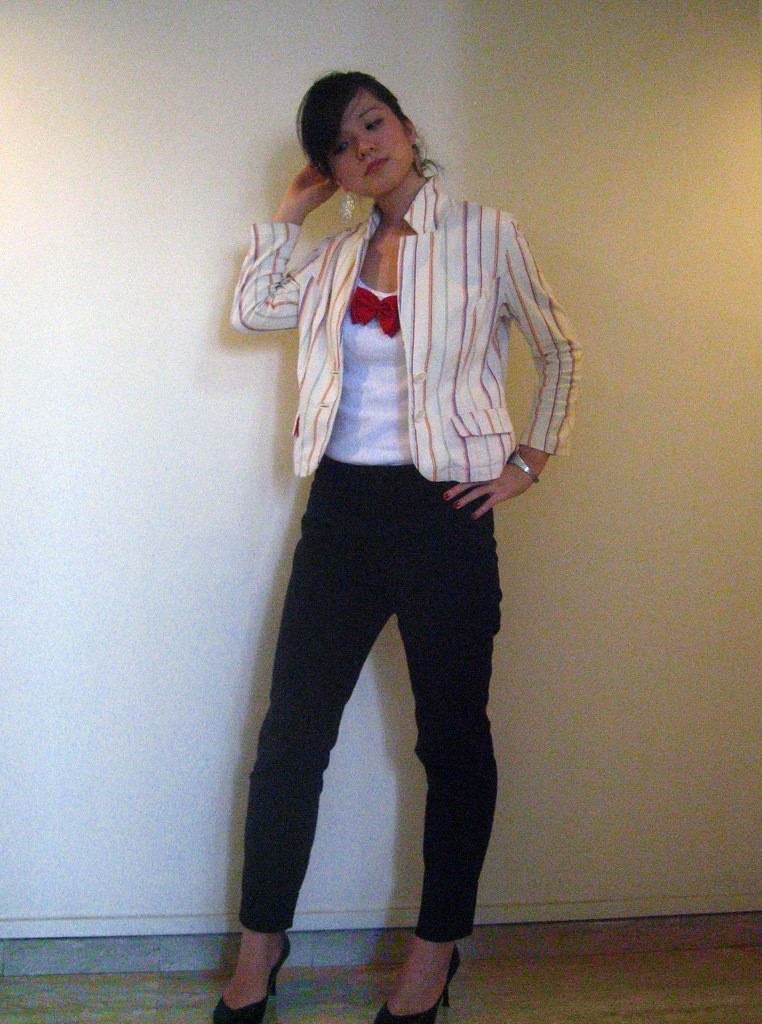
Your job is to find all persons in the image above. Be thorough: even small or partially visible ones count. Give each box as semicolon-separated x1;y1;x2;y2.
209;68;576;1023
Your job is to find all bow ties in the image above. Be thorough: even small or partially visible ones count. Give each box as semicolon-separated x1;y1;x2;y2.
350;286;400;338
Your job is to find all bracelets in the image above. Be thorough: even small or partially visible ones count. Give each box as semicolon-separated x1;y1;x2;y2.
505;448;543;486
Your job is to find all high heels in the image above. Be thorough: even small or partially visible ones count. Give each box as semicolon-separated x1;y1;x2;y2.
374;944;460;1024
213;932;290;1024
